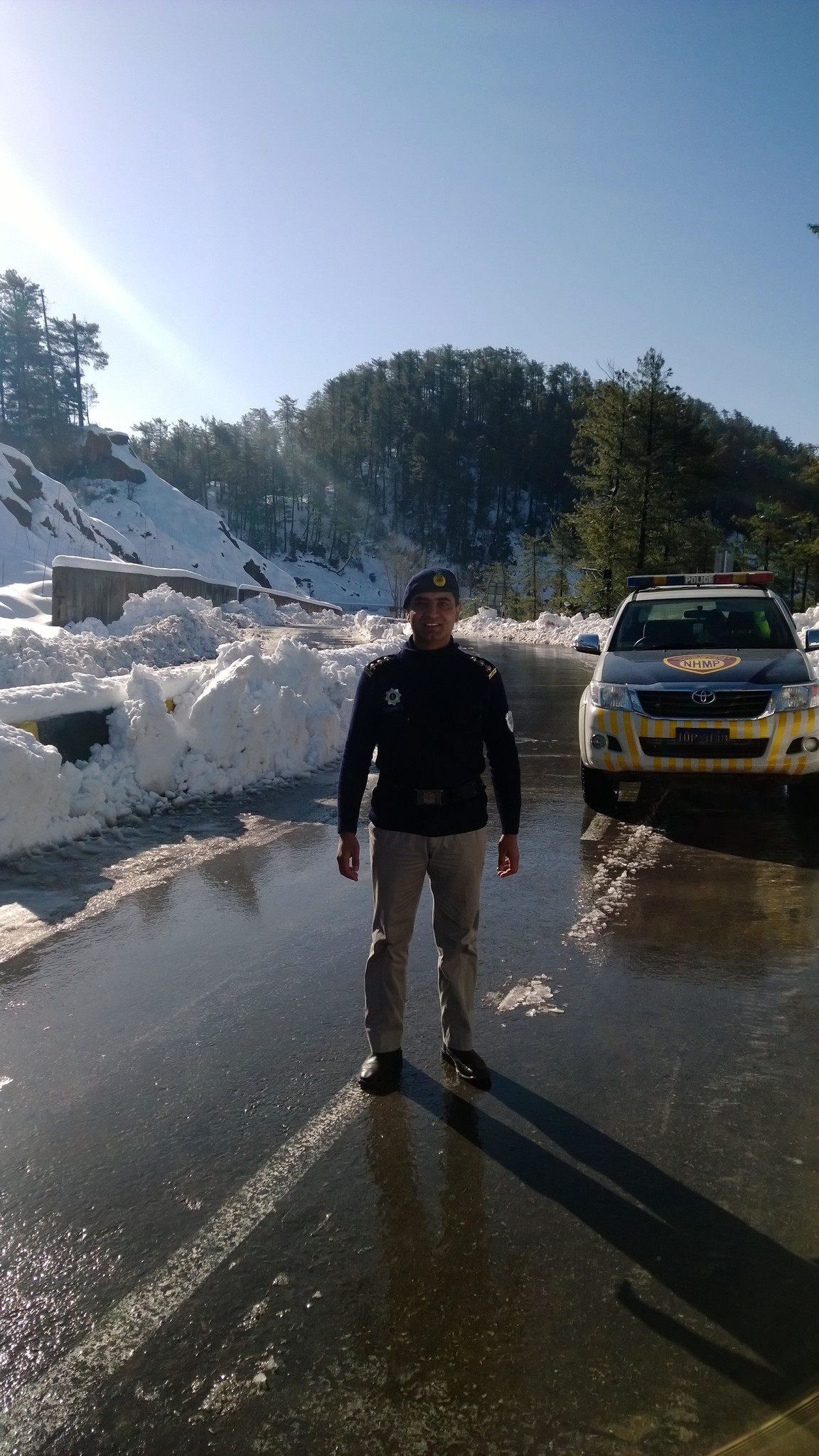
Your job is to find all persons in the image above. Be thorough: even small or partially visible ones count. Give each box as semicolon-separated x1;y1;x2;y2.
337;567;521;1085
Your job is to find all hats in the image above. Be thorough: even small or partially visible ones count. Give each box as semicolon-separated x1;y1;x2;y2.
403;567;459;611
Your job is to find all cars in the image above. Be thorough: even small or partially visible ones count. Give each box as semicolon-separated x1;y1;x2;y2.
571;570;819;807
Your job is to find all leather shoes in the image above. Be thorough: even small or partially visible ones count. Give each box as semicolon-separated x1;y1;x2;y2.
358;1045;405;1086
440;1037;489;1083
